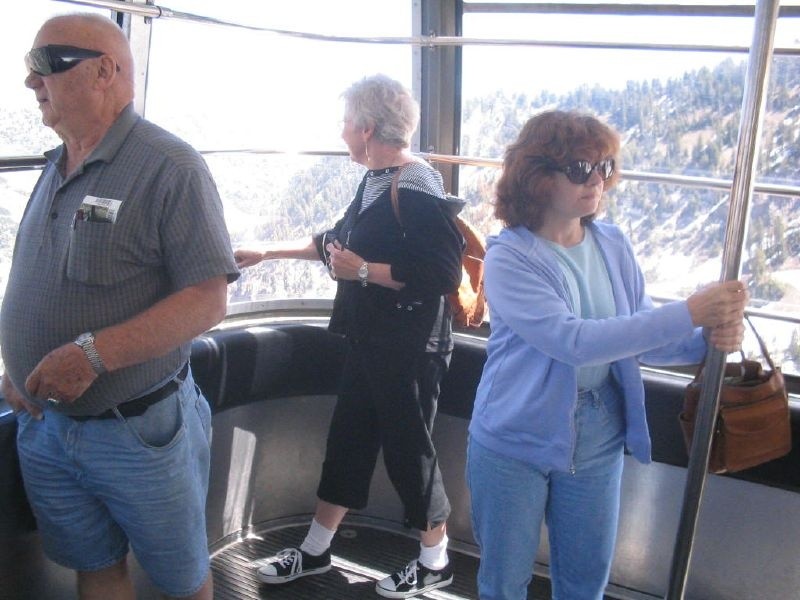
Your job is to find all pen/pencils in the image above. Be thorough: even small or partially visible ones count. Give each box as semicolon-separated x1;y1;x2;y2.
334;241;345;280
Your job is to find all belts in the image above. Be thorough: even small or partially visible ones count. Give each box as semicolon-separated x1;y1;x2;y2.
70;362;188;422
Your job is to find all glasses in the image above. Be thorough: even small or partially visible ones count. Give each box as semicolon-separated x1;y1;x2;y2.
24;42;119;75
551;158;615;184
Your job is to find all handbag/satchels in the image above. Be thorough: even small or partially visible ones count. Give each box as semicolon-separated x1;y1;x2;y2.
680;306;791;474
391;161;491;327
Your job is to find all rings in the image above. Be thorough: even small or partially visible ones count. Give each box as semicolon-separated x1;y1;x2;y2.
48;399;59;408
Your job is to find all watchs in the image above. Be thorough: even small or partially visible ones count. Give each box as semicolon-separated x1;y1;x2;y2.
74;332;107;375
358;261;368;287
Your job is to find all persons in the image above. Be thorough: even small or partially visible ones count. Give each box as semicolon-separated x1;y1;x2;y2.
232;74;467;599
0;14;241;600
464;110;749;599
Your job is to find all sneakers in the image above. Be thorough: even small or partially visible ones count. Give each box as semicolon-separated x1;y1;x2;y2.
256;545;332;584
376;559;454;598
